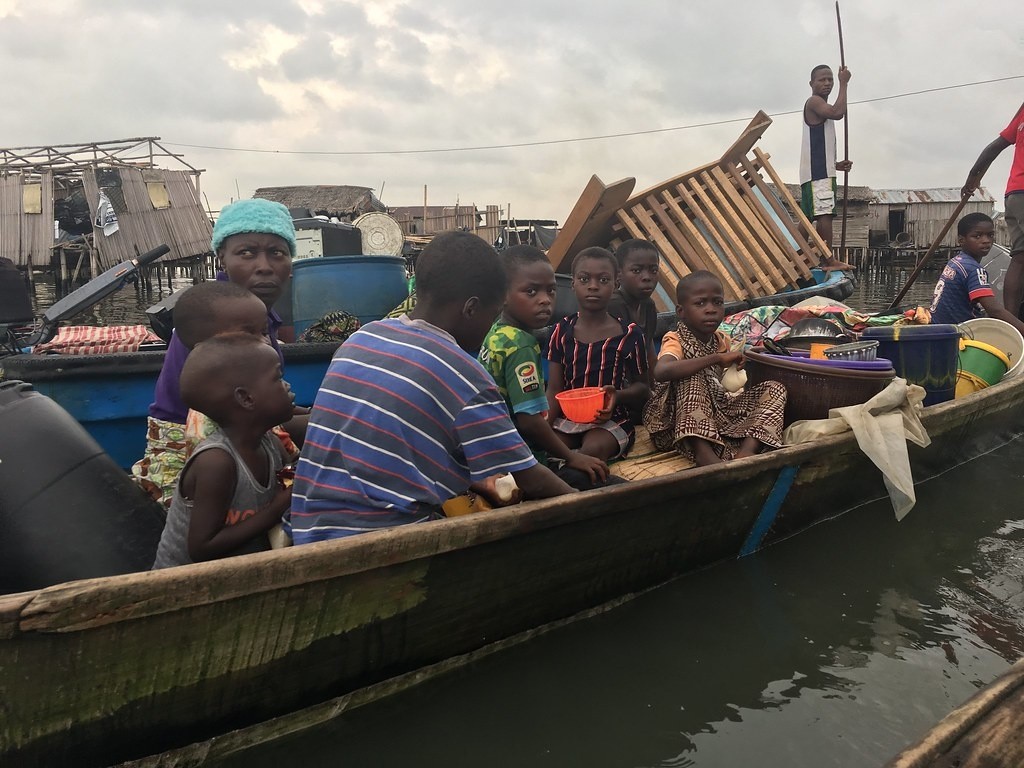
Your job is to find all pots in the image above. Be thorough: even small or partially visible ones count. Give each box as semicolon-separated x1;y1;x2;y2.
774;318;849;347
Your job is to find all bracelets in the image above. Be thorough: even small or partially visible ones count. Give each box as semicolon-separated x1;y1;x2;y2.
305;407;311;414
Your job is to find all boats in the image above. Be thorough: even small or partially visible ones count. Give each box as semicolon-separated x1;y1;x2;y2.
1;196;853;475
882;655;1024;767
0;316;1024;767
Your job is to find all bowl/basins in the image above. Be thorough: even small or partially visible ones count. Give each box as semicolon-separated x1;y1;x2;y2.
823;339;879;361
556;387;603;423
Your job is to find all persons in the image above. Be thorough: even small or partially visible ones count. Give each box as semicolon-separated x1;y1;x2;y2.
961;101;1024;321
797;64;857;272
132;198;314;570
476;238;669;493
930;212;1024;338
283;230;580;549
643;270;786;469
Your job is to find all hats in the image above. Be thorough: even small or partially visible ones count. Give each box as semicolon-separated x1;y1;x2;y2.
210;197;298;261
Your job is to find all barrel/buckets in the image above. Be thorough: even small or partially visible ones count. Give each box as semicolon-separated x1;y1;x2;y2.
744;318;1024;431
292;255;407;344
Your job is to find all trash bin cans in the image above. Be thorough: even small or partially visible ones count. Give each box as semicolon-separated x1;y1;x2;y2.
290;255;411;340
